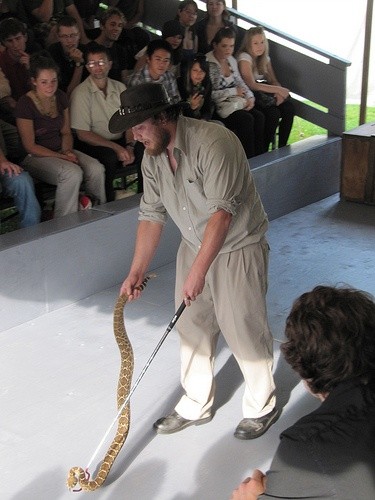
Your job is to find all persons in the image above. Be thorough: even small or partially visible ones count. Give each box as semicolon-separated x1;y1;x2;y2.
107;82;282;440
232;283;375;500
0;0;297;234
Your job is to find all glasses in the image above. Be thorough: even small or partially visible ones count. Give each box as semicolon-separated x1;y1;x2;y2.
192;55;205;61
86;59;107;68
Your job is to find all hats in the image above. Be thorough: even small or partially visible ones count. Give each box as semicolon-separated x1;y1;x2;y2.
162;22;184;39
108;84;189;133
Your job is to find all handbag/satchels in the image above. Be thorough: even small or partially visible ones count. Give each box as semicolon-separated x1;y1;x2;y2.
256;82;291;108
217;96;248;119
79;195;92;211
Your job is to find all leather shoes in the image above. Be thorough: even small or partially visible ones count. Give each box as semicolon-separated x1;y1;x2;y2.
153;411;212;434
234;408;279;439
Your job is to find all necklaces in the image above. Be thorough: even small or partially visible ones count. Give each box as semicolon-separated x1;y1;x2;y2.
28;86;56;118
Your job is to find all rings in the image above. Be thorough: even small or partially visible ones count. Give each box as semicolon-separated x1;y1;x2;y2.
183;296;189;301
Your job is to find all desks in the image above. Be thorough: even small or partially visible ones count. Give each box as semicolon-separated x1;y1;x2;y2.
337;123;375;207
